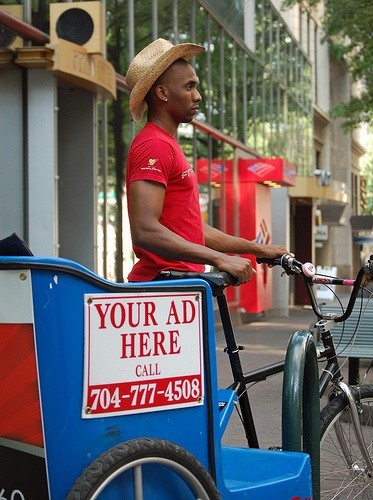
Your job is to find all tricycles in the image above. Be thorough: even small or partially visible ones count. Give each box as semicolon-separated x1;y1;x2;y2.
0;252;373;500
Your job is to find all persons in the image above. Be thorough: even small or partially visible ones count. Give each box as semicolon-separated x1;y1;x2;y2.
125;38;293;286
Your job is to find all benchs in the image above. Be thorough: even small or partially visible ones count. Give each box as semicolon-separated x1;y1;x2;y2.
308;298;373;385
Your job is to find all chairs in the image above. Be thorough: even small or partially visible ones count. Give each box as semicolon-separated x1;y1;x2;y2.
0;258;313;500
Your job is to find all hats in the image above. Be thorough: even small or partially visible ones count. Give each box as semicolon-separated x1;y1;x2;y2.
125;38;206;122
